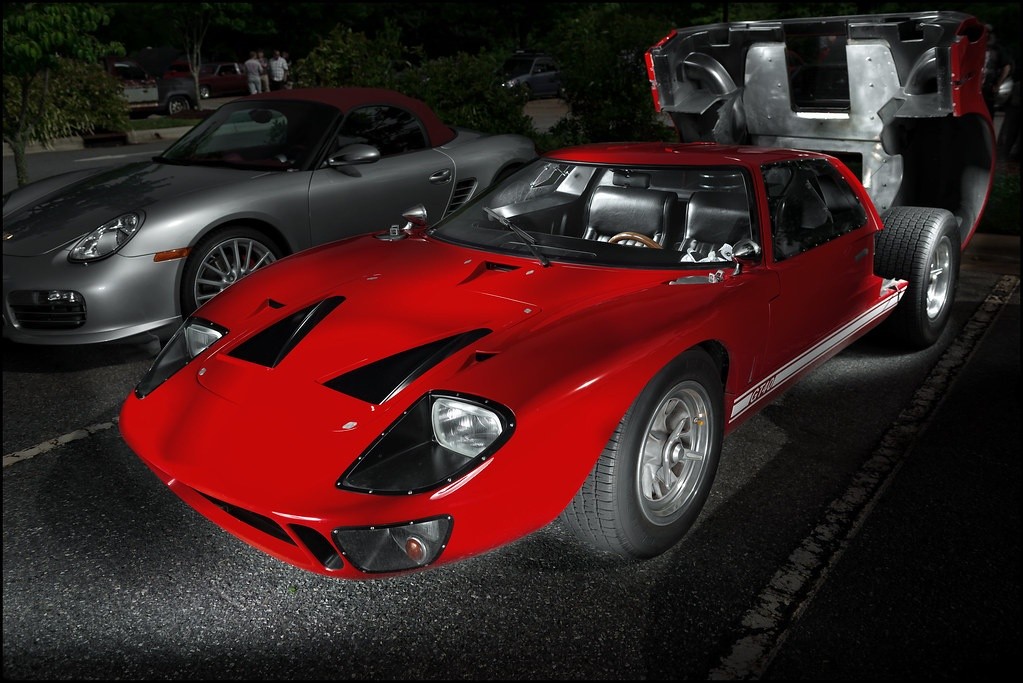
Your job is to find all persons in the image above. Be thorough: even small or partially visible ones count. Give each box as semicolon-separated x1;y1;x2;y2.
257;52;270;92
984;24;998;47
244;51;263;95
990;45;1015;109
267;49;288;91
281;51;292;84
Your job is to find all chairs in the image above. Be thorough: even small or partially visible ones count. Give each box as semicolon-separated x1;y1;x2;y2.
678;189;803;259
582;185;680;249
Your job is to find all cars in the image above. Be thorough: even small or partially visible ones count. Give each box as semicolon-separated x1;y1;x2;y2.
111;58;198;116
162;61;252;99
496;51;574;102
996;76;1012;108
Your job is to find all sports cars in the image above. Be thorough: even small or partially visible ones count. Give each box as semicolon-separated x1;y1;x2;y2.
3;88;540;348
119;11;1015;581
